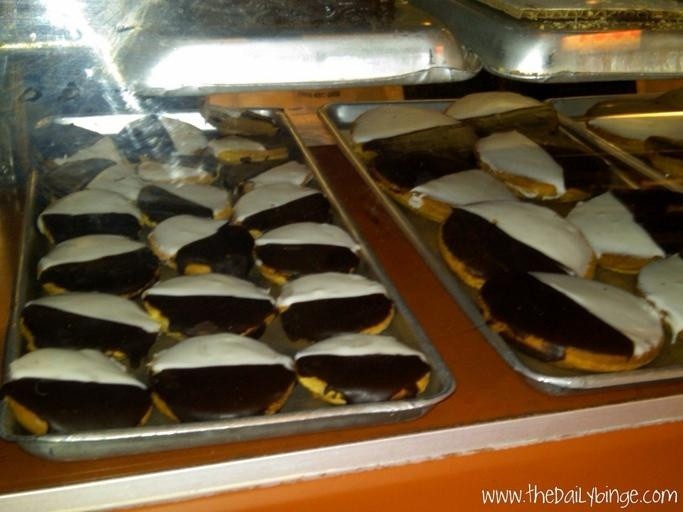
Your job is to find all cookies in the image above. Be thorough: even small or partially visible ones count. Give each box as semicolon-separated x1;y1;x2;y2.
2;103;432;439
355;90;682;383
